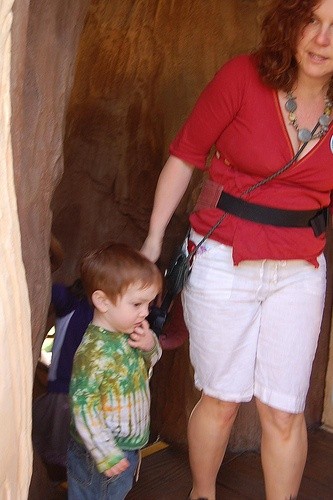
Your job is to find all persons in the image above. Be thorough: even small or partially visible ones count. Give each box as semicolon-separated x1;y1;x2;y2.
139;1;333;500
65;243;163;500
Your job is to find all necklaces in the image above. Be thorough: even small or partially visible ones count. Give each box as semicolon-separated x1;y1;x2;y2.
284;77;332;143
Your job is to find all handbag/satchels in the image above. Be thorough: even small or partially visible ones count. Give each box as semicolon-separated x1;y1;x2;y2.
37;279;169;391
163;247;187;295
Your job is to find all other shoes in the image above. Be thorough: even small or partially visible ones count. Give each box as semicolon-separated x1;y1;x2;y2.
186;487;208;500
